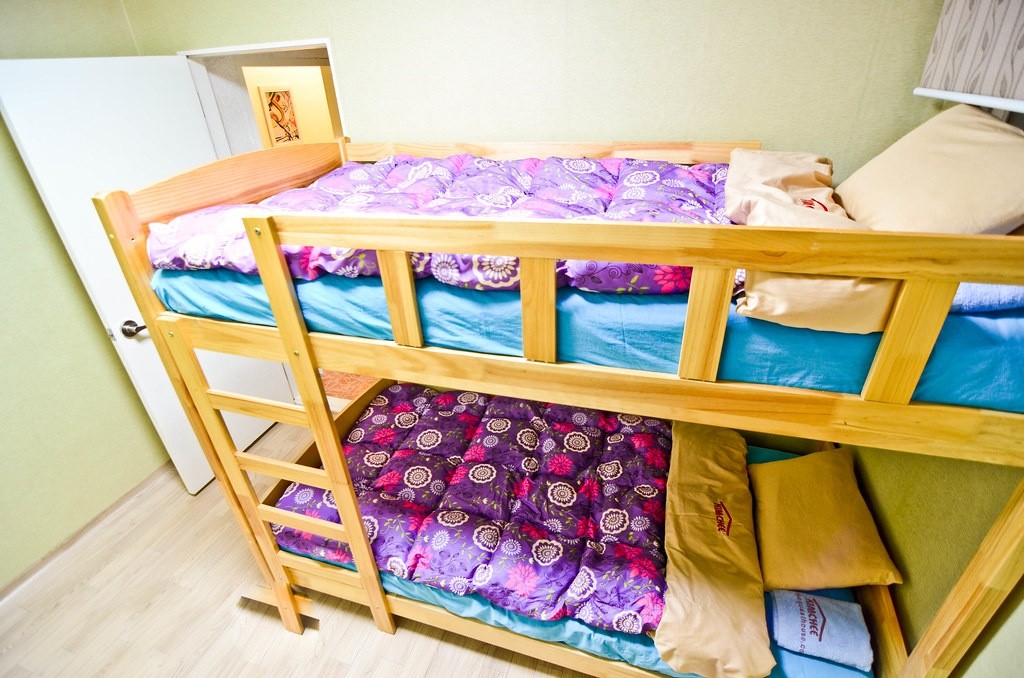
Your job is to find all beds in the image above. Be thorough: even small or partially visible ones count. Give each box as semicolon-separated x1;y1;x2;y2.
93;136;1024;678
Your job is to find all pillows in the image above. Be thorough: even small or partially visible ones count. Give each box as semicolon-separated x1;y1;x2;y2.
749;447;903;592
833;104;1024;236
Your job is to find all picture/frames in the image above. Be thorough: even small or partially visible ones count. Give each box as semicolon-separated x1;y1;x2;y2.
258;86;304;147
